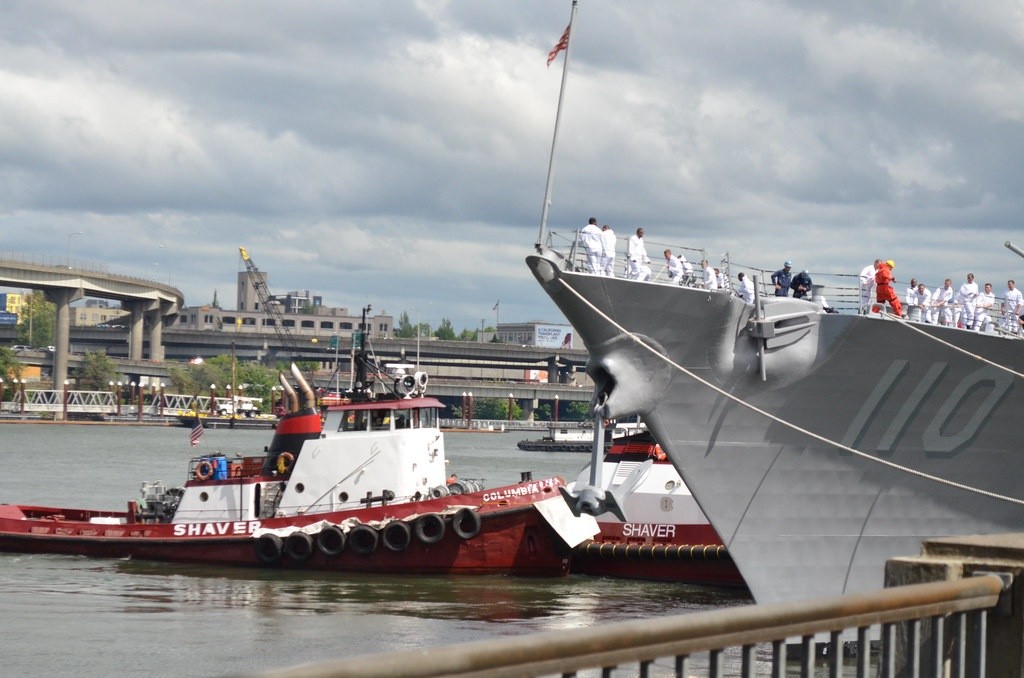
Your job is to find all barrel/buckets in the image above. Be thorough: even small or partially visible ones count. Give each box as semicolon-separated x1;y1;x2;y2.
199;457;227;479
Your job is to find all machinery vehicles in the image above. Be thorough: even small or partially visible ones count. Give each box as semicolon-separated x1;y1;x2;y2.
216;395;263;418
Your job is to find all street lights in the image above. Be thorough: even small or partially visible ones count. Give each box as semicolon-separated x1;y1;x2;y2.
271;386;276;415
237;385;243;396
411;307;420;371
0;377;27;416
508;393;514;426
468;391;473;430
210;384;216;417
461;392;467;427
64;380;69;421
109;381;165;423
280;385;286;408
226;384;232;398
553;395;560;427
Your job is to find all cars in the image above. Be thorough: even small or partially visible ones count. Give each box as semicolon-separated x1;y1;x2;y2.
10;345;56;351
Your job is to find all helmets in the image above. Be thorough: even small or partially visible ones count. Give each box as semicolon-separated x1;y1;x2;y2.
802;269;809;280
784;260;792;268
886;260;895;268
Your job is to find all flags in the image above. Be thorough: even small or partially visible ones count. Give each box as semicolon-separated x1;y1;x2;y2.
547;23;571;68
190;410;204;446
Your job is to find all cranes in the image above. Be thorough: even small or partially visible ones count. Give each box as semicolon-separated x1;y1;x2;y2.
238;245;316;391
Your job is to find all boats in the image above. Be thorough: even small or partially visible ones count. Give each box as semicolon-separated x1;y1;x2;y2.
565;430;750;588
174;401;281;430
517;422;649;452
525;228;1024;658
0;395;568;576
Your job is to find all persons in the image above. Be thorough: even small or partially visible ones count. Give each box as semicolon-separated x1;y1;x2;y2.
579;218;812;304
447;474;457;486
858;259;1024;338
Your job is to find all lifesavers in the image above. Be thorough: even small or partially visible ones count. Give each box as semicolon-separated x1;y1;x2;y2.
347;525;380;557
317;527;346;555
254;533;283;563
382;520;413;551
451;507;481;540
588;444;593;451
569;445;575;451
547;445;553;451
414;513;445;543
605;446;611;452
655;443;667;461
561;444;567;451
579;444;586;451
196;461;214;481
526;445;531;450
283;532;315;561
276;452;295;474
